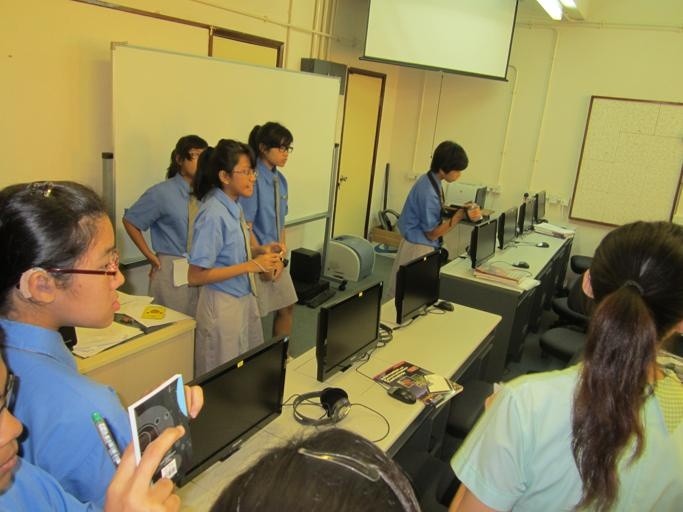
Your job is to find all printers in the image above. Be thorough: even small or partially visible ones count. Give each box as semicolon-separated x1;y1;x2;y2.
324;234;375;284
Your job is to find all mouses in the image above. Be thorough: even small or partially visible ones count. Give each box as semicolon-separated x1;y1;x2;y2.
437;302;453;311
387;386;415;404
536;242;548;247
512;261;530;269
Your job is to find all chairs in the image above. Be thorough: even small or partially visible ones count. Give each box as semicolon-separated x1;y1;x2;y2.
539;255;593;363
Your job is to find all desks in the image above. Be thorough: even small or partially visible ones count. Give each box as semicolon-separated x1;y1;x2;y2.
56;290;197;404
439;204;576;380
160;283;503;512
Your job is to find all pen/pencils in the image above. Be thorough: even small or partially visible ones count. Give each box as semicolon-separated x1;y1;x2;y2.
91;412;122;467
276;251;284;259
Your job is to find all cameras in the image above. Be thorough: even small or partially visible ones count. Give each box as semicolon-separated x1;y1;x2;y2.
270;246;288;267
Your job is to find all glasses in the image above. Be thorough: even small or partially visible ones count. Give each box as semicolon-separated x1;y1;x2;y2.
233;169;258;176
48;253;120;276
278;143;293;153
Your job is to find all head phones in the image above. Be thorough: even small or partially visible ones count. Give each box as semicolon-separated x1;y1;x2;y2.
378;321;392;344
292;387;351;426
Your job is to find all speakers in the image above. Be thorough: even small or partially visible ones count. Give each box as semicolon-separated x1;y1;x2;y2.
289;248;321;305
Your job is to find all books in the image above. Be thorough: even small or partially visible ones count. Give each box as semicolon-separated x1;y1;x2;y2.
533;223;575;239
374;361;464;407
475;263;540;291
128;374;192;482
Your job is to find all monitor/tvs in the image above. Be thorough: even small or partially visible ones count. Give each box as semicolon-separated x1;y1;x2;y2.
470;218;497;269
519;198;536;235
496;207;518;249
394;249;441;324
534;191;548;224
176;334;289;489
315;279;383;383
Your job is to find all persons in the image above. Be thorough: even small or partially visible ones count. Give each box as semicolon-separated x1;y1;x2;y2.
237;122;299;368
210;430;419;511
1;328;204;511
392;142;482;275
0;181;132;500
122;135;208;317
449;222;683;512
188;139;287;379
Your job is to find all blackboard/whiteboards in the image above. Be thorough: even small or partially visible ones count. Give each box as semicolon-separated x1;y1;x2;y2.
109;41;342;271
568;95;683;228
